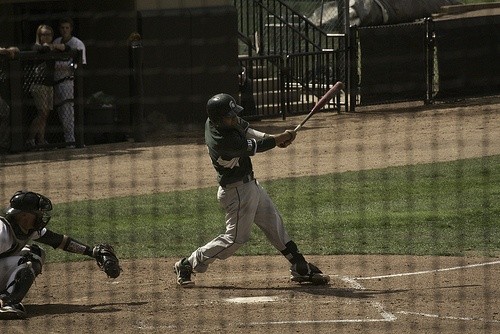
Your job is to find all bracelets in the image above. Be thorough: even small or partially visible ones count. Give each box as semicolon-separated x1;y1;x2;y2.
285;130;293;140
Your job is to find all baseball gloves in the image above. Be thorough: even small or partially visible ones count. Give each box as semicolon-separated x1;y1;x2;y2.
91;242;122;279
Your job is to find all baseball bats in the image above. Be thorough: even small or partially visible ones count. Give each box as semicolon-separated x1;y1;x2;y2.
278;81;344;149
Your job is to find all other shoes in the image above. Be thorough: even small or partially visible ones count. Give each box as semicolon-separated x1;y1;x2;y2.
0;298;25;319
22;141;60;152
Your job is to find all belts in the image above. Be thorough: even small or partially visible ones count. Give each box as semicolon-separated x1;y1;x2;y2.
243;171;255;184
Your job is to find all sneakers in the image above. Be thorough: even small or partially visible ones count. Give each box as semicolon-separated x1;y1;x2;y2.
290;260;330;284
173;258;196;288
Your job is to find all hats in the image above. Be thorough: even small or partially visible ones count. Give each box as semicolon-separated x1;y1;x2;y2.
10;190;40;214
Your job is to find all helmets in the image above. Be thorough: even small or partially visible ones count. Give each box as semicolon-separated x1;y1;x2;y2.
207;93;244;119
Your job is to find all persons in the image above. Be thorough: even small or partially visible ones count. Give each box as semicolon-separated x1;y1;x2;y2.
0;46;20;146
23;24;71;147
173;93;330;288
52;17;86;143
0;191;110;320
